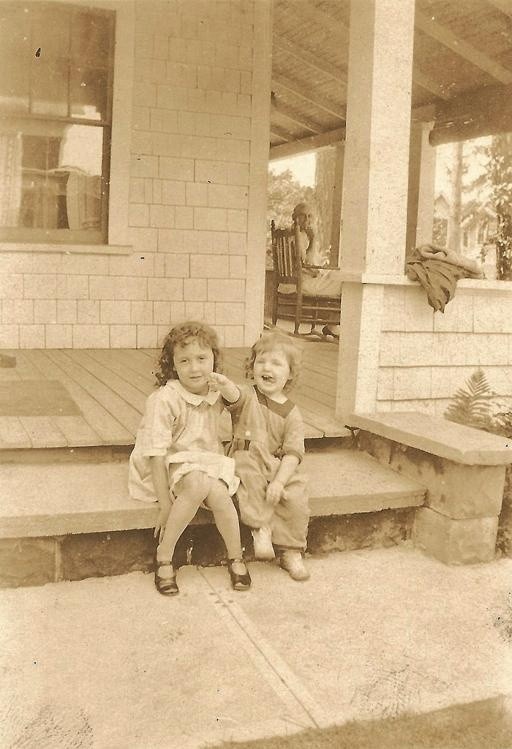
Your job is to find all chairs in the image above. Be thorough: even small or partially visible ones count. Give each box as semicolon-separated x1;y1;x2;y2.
270;220;341;340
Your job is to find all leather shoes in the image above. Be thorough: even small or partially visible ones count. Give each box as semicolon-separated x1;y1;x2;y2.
227;557;251;591
154;561;180;597
321;326;339;340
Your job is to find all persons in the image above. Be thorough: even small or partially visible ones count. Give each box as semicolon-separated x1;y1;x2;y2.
206;329;315;582
121;319;255;597
275;203;348;342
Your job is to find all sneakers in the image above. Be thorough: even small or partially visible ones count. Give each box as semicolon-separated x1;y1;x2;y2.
253;526;277;563
279;550;311;580
